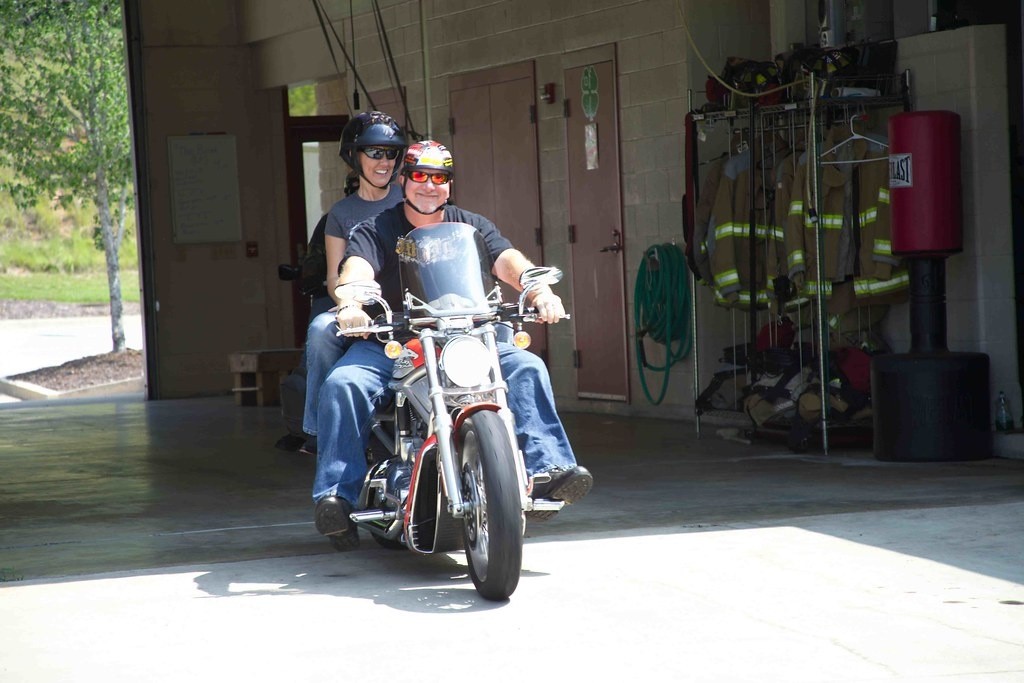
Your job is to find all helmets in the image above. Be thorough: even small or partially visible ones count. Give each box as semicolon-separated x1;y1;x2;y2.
339;111;407;156
707;48;852;103
401;140;454;174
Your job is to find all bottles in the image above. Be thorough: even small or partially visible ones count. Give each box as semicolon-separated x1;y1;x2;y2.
996;391;1014;432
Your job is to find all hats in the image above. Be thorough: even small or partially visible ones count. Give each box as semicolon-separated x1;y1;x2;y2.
344;170;359;193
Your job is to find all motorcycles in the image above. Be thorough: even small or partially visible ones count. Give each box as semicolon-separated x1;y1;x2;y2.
333;222;573;601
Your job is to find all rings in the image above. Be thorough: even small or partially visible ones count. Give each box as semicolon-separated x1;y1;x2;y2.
346;323;352;328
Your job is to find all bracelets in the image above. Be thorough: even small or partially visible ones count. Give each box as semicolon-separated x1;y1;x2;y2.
338;304;354;315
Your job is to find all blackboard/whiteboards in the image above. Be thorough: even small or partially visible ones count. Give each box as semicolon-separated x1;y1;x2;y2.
167;133;242;246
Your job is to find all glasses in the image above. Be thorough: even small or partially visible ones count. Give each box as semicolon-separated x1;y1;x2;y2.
404;170;450;185
361;147;398;159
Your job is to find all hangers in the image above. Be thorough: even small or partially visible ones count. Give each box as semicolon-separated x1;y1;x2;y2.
817;113;889;164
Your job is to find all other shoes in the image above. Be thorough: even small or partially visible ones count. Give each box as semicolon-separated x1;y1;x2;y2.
298;440;316;455
275;435;303;451
529;467;593;522
315;496;360;551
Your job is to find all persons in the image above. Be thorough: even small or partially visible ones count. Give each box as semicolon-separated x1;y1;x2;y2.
303;111;406;435
311;140;593;551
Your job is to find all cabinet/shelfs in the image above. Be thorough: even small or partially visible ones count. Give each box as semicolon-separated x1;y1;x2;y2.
689;69;915;453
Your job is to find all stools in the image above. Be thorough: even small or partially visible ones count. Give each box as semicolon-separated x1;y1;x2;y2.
227;348;303;408
